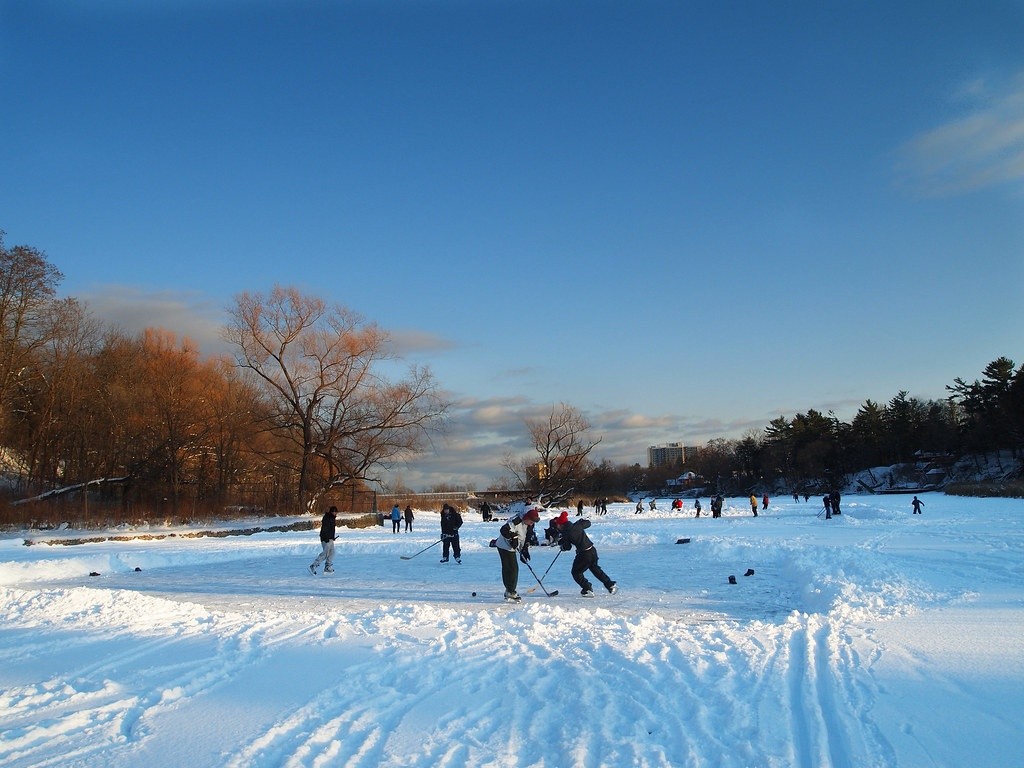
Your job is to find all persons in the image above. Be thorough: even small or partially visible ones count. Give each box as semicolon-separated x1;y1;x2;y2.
555;512;618;598
600;496;608;515
495;509;540;604
823;489;841;519
694;493;724;518
762;494;768;510
750;492;758;517
404;506;414;534
308;506;338;577
542;517;560;546
391;504;401;534
670;498;683;511
649;499;657;511
912;496;925;514
593;496;601;515
520;496;536;548
440;504;463;564
635;499;644;514
576;497;583;516
478;502;494;522
792;491;810;503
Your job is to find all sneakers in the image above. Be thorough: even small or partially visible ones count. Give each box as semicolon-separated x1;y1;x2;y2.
581;587;595;597
455;556;462;564
608;580;620;596
440;556;449;564
307;561;320;576
324;563;334;575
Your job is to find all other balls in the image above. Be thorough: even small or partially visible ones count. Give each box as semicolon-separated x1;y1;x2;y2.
472;592;476;597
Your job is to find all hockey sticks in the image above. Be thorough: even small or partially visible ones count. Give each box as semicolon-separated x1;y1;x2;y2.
400;536;448;560
517;547;559;598
525;550;561;593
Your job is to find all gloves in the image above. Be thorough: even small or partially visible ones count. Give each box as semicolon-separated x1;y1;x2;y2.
508;533;519;549
560;542;572;551
450;527;458;538
441;532;449;542
519;546;530;563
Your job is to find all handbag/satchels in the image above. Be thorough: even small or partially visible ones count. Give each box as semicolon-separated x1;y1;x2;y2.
489;539;498;547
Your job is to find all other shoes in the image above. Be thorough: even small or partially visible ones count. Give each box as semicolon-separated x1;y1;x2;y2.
744;568;754;577
728;575;737;584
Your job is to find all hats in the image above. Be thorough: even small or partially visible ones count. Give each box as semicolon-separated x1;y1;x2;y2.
329;505;338;512
556;511;569;525
526;508;540;522
443;503;450;510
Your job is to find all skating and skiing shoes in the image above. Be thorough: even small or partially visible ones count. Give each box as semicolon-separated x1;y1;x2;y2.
504;589;522;604
